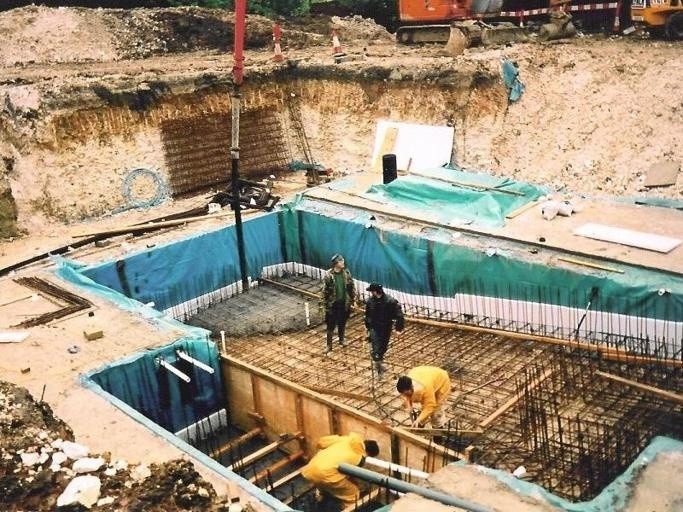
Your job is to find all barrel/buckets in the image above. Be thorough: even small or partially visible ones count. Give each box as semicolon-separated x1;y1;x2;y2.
383;154;397;185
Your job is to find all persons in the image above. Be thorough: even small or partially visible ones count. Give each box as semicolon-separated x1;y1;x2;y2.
322;253;356;354
300;432;379;512
365;282;404;382
397;364;451;444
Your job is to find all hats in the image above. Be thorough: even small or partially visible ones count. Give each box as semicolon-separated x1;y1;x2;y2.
365;283;382;291
330;254;343;264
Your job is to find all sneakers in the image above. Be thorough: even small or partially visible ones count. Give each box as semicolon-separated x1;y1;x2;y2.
322;346;332;354
339;341;348;347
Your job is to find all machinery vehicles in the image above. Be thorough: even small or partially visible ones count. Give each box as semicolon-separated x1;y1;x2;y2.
629;0;682;41
396;1;528;59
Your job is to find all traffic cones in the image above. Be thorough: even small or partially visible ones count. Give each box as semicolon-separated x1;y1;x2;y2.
519;10;524;26
612;7;620;34
273;37;284;64
332;29;346;57
